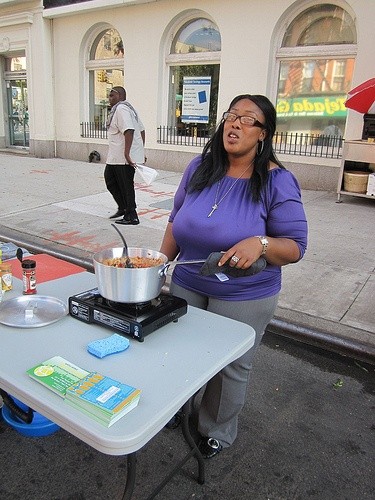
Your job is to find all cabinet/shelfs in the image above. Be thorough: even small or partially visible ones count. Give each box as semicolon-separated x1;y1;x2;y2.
335;139;375;206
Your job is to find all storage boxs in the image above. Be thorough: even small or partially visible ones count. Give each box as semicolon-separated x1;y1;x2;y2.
365;172;375;197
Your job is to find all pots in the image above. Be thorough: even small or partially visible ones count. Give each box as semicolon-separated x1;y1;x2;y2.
93;247;207;303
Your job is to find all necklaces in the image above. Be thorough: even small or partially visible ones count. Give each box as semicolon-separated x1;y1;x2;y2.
207;158;256;218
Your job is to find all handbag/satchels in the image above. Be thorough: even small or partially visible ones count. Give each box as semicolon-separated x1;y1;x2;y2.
133;164;157;185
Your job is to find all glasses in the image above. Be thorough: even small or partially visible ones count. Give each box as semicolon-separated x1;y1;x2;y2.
110;88;118;93
222;112;265;129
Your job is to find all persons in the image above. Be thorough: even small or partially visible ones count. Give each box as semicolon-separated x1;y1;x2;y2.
104;86;147;225
321;120;343;146
157;94;308;459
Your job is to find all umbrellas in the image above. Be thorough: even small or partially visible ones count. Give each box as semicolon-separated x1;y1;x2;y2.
343;77;375;114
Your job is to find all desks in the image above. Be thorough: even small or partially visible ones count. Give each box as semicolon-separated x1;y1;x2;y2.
0;240;256;500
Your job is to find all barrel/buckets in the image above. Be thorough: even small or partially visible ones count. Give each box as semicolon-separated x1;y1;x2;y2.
344;171;369;193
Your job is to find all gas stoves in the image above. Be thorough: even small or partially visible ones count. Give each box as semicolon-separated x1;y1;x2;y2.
68;287;188;343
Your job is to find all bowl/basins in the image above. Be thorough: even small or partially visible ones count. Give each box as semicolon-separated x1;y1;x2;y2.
2;394;61;437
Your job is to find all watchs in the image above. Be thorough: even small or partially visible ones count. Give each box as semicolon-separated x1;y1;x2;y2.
254;236;269;256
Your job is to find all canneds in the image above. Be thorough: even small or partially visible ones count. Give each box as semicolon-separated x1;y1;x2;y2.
0;262;14;291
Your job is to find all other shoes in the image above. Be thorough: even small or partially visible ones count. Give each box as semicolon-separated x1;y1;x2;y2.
199;437;223;460
115;218;139;225
110;209;127;218
165;408;184;430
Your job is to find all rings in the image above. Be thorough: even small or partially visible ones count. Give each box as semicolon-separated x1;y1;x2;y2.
232;256;239;262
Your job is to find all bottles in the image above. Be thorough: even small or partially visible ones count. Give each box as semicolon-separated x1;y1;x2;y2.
16;248;37;294
